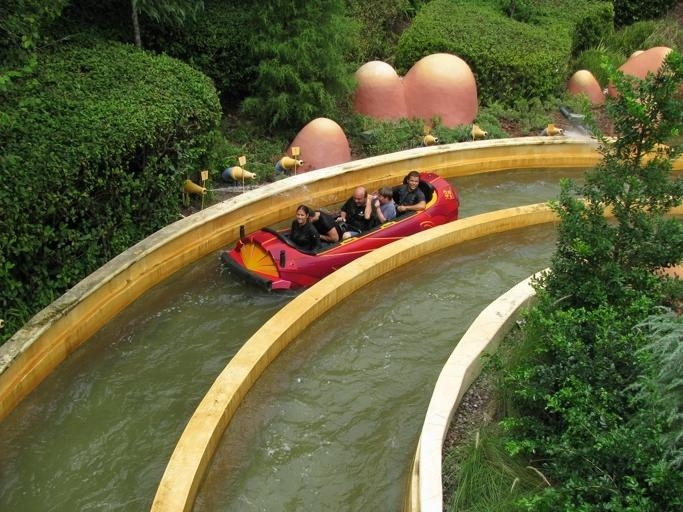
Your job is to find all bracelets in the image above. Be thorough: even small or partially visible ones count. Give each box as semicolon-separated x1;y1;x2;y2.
374;205;380;210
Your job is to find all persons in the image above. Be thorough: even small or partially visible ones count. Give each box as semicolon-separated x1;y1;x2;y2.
335;186;376;240
308;209;343;243
288;205;319;254
388;171;425;215
373;186;397;223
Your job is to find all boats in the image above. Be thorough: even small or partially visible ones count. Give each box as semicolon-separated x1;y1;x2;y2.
221;172;459;294
